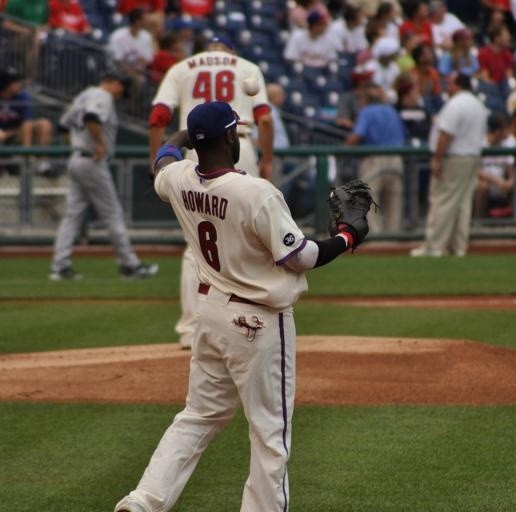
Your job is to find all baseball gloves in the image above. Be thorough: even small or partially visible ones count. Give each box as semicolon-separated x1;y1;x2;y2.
328;179;371;249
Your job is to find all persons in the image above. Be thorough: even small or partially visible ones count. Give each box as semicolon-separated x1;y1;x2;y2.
406;71;489;260
144;22;277;350
108;97;376;512
345;86;406;240
47;71;159;284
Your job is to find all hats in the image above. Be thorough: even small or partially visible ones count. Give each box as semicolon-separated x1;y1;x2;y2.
307;9;329;26
205;36;236;50
187;102;249;140
101;68;132;98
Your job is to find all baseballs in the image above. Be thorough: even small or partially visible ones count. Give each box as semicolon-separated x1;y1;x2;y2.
244;78;260;95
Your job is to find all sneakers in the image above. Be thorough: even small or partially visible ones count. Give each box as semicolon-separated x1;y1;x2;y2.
180;338;191;349
410;247;466;257
49;266;84;281
119;263;159;280
